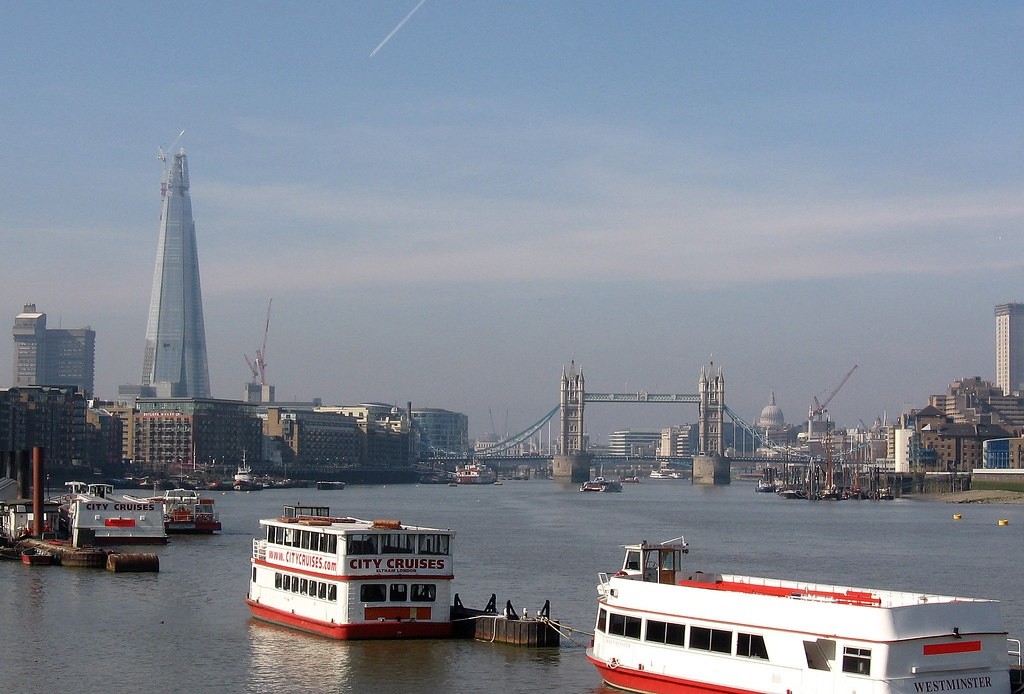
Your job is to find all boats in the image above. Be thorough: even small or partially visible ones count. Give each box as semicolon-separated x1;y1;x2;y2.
618;475;639;483
21;547;52;566
243;501;453;641
1;475;172;568
754;465;894;501
455;463;497;484
669;472;683;479
579;477;623;493
208;470;263;491
316;481;345;491
650;469;671;479
145;489;221;534
582;537;1024;693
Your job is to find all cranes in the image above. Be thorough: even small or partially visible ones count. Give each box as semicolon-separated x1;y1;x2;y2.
244;297;272;385
813;364;858;447
